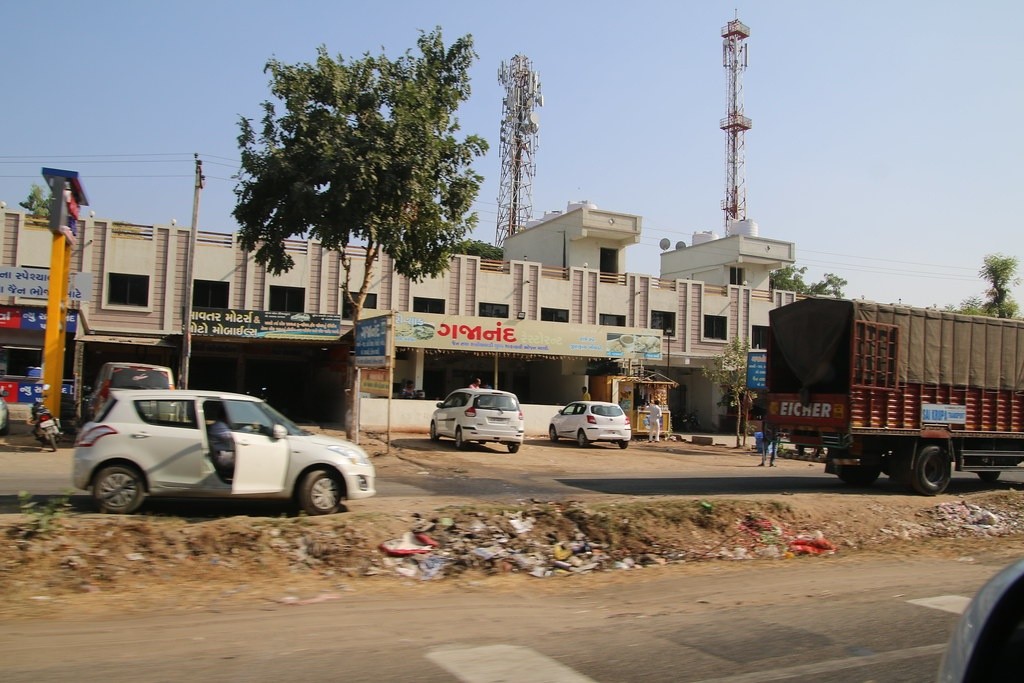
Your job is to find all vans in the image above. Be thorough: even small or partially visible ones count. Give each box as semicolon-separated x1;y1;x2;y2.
86;360;176;424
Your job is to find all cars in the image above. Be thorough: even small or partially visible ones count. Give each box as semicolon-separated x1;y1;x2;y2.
0;389;11;437
548;400;633;449
71;387;377;516
430;388;525;454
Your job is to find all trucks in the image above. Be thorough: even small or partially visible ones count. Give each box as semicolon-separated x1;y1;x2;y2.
761;295;1024;496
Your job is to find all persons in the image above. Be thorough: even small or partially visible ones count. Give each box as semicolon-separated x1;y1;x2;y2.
467;378;481;399
580;387;591;401
209;404;264;466
759;417;782;468
648;400;662;443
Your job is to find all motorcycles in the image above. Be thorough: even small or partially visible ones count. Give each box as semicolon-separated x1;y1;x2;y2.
27;384;64;451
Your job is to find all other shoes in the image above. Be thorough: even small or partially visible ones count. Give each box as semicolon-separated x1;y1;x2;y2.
655;441;661;443
648;441;652;443
770;464;777;467
758;464;765;466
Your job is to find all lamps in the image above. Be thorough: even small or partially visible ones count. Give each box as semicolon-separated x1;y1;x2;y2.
517;312;526;320
